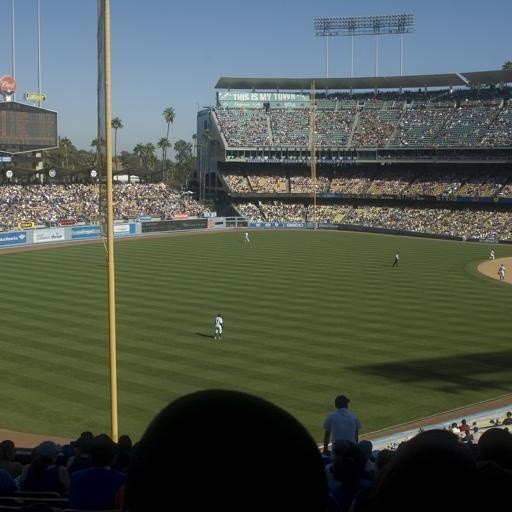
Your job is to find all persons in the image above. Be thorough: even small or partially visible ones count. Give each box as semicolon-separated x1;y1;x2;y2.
0;180;217;232
488;249;495;260
214;314;224;340
0;389;512;512
211;90;512;244
499;264;506;279
392;251;400;267
